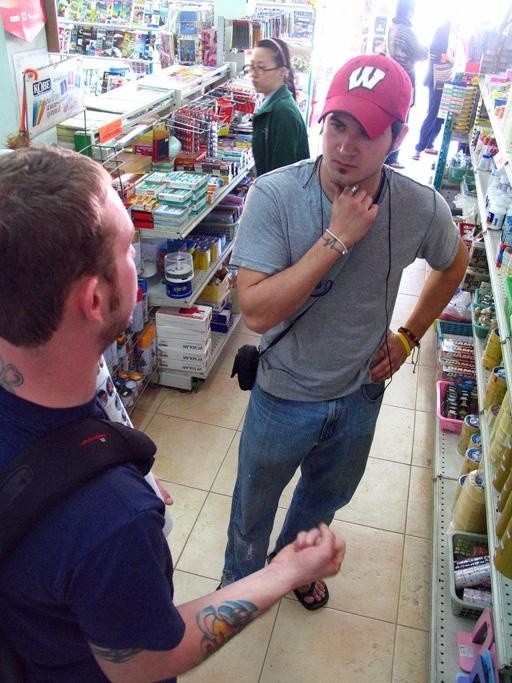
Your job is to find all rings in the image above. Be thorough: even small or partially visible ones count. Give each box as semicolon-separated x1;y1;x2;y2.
344;184;357;192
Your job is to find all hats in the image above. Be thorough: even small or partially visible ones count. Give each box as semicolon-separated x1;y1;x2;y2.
316;52;413;138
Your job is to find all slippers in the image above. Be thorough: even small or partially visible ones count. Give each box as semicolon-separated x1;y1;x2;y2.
293;581;330;612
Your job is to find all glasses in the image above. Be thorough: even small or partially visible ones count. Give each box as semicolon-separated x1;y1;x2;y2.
246;62;283;74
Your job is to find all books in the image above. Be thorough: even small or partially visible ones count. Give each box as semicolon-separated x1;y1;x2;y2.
53;0;292;143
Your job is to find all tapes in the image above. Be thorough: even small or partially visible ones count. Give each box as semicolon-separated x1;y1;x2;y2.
452;328;512;580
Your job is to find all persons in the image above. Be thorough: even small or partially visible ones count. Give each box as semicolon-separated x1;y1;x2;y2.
246;34;311;174
386;0;430;168
409;11;470;157
213;54;468;612
0;143;347;682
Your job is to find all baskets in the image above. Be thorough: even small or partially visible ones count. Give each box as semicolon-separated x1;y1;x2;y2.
438;320;474;336
458;221;485;244
437;379;473;434
446;528;496;620
199;214;242;240
463;175;475;191
473;287;498;336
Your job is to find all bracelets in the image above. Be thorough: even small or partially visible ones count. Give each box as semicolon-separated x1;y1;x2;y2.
394;330;411;355
325;228;348;253
398;325;421;348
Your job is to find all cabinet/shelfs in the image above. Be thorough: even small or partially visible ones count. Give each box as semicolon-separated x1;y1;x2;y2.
1;1;512;683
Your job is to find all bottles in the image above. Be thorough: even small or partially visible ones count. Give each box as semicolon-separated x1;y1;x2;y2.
485;175;511;232
130;278;150;334
113;370;143;408
137;323;156;378
478;154;491;171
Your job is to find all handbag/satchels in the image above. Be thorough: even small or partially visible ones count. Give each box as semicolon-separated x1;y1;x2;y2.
230;342;258;392
